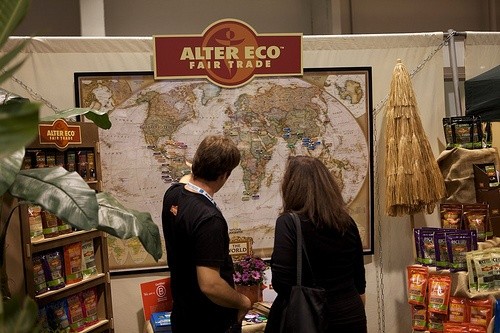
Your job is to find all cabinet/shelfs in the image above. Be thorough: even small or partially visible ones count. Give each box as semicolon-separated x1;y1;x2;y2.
0;120;114;333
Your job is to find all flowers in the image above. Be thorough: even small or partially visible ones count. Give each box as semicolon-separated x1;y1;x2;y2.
233;255;267;286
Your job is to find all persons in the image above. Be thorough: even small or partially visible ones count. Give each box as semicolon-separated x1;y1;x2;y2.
161;136;252;333
269;154;368;333
